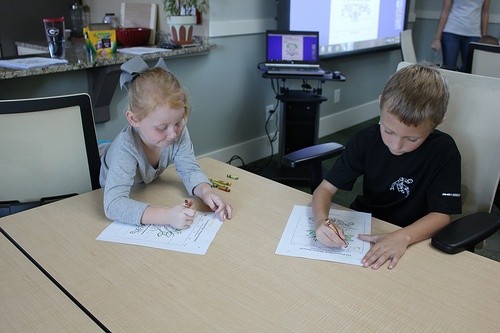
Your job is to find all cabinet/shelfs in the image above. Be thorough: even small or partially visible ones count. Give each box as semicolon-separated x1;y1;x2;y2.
275;90;327;193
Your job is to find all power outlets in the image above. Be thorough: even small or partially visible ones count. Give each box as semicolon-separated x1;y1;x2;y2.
266;104;275;121
333;89;340;103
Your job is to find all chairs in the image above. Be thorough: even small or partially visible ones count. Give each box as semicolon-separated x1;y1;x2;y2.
280;62;499;254
464;42;500;77
399;29;417;63
0;93;102;216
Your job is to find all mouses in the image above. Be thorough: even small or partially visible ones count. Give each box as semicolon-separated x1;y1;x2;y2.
333;71;341;79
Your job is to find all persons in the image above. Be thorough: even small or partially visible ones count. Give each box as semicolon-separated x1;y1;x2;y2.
312;63;463;270
431;0;490;74
99;56;234;230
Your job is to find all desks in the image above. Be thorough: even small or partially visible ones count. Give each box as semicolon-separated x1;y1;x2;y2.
0;156;499;333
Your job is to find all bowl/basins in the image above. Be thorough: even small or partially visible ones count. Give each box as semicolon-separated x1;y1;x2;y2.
116;28;151;47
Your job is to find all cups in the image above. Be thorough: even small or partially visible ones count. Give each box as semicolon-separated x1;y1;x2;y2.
43;17;65;60
65;30;72;41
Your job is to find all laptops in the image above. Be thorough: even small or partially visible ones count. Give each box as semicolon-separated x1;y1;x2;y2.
266;29;325;75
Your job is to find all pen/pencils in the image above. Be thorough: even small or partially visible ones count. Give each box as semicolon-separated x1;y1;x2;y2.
208;179;231;192
185;200;189;207
325;219;348;246
227;174;238;180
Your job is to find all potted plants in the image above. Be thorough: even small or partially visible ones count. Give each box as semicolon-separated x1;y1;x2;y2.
162;0;209;47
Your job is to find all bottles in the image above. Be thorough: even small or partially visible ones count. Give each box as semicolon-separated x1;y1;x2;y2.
71;3;83;37
